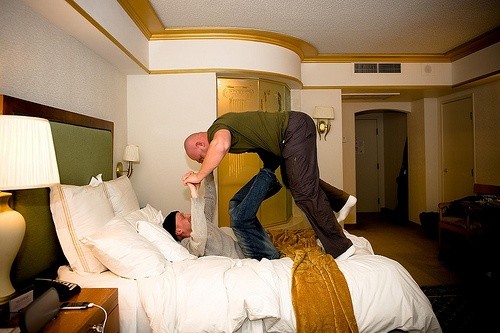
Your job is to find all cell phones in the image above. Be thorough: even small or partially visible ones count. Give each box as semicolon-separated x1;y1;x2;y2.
59;302;90;309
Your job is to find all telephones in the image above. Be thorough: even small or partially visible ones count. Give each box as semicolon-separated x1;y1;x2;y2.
33;280;80;298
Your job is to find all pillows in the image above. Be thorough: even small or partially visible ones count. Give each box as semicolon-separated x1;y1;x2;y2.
124;203;158;231
49;184;114;273
80;211;168;280
135;210;190;263
96;174;140;216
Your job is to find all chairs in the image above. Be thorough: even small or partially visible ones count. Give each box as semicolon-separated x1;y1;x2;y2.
438;184;497;239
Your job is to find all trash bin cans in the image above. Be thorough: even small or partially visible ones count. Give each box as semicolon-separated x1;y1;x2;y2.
419;212;439;240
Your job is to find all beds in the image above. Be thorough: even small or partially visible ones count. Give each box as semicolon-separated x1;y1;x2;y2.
0;95;442;333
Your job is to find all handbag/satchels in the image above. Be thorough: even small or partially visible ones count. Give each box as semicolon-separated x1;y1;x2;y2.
420;212;440;240
421;285;464;333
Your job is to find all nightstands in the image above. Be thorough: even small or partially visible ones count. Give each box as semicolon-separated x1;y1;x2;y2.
44;288;120;333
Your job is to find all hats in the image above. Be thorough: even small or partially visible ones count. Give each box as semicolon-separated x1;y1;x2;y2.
163;211;179;241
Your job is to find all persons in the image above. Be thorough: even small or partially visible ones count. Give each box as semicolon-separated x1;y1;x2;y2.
183;110;357;262
162;148;284;262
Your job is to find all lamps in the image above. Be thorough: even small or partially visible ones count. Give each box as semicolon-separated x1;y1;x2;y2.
116;144;140;178
0;115;60;298
311;105;335;141
